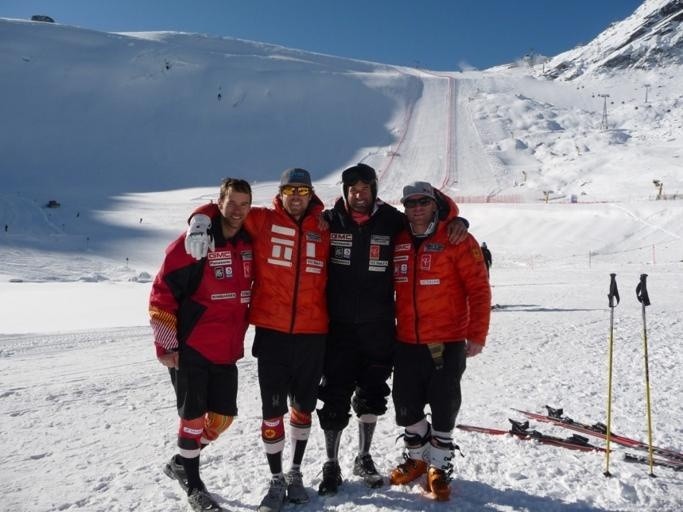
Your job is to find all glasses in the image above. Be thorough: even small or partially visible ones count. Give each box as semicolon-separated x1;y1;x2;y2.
280;185;310;196
401;196;431;208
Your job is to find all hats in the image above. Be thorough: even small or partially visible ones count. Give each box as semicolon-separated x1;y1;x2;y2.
341;163;377;184
278;167;315;190
399;181;437;203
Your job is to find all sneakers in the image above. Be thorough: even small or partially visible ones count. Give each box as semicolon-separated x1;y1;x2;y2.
352;454;386;489
282;470;309;504
163;453;190;494
388;451;429;486
316;460;343;497
256;476;286;512
427;463;451;504
185;482;223;512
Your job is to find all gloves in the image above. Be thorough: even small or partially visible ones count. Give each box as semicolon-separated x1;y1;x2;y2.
184;214;216;261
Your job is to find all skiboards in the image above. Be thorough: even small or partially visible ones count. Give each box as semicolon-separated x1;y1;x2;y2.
457;408;683;470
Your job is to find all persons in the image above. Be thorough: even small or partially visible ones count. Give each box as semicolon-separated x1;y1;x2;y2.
148;176;256;512
186;165;329;512
481;241;492;278
317;162;470;497
391;180;492;501
